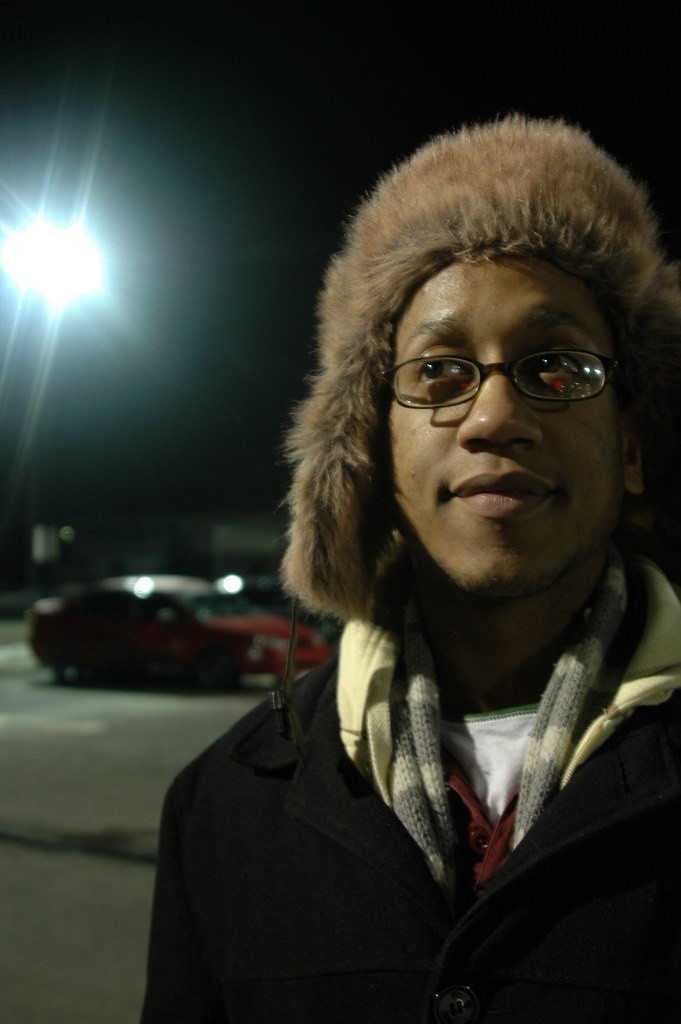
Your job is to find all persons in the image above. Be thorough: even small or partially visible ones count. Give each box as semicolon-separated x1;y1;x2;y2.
140;116;681;1024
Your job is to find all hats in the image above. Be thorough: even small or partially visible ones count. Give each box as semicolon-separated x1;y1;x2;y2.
276;119;681;620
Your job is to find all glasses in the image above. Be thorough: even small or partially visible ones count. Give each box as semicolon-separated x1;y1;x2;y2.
382;348;619;410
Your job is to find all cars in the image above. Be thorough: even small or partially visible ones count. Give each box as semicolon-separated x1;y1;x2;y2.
27;575;332;695
